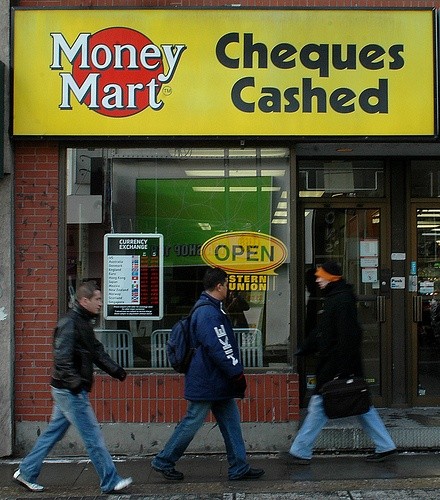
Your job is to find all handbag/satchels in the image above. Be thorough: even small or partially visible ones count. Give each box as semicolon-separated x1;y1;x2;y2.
319;373;369;419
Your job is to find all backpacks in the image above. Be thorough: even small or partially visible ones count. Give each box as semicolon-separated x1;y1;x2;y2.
165;302;223;373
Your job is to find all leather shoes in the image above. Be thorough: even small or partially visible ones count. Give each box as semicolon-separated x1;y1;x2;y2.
152;463;183;480
228;468;265;480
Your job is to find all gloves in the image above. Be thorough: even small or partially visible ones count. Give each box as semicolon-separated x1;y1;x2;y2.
70;385;82;395
232;372;246;393
116;368;127;380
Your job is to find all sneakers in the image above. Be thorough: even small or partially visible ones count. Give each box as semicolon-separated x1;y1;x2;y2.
101;477;133;494
12;468;44;491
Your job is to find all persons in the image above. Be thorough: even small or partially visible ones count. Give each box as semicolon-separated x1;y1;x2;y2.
151;267;265;481
222;288;250;347
305;268;321;336
12;282;135;493
280;258;399;465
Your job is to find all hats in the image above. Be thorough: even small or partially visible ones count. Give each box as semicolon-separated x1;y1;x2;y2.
314;261;342;281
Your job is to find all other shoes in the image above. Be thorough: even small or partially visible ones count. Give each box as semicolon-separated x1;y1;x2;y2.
367;449;397;462
288;453;311;464
